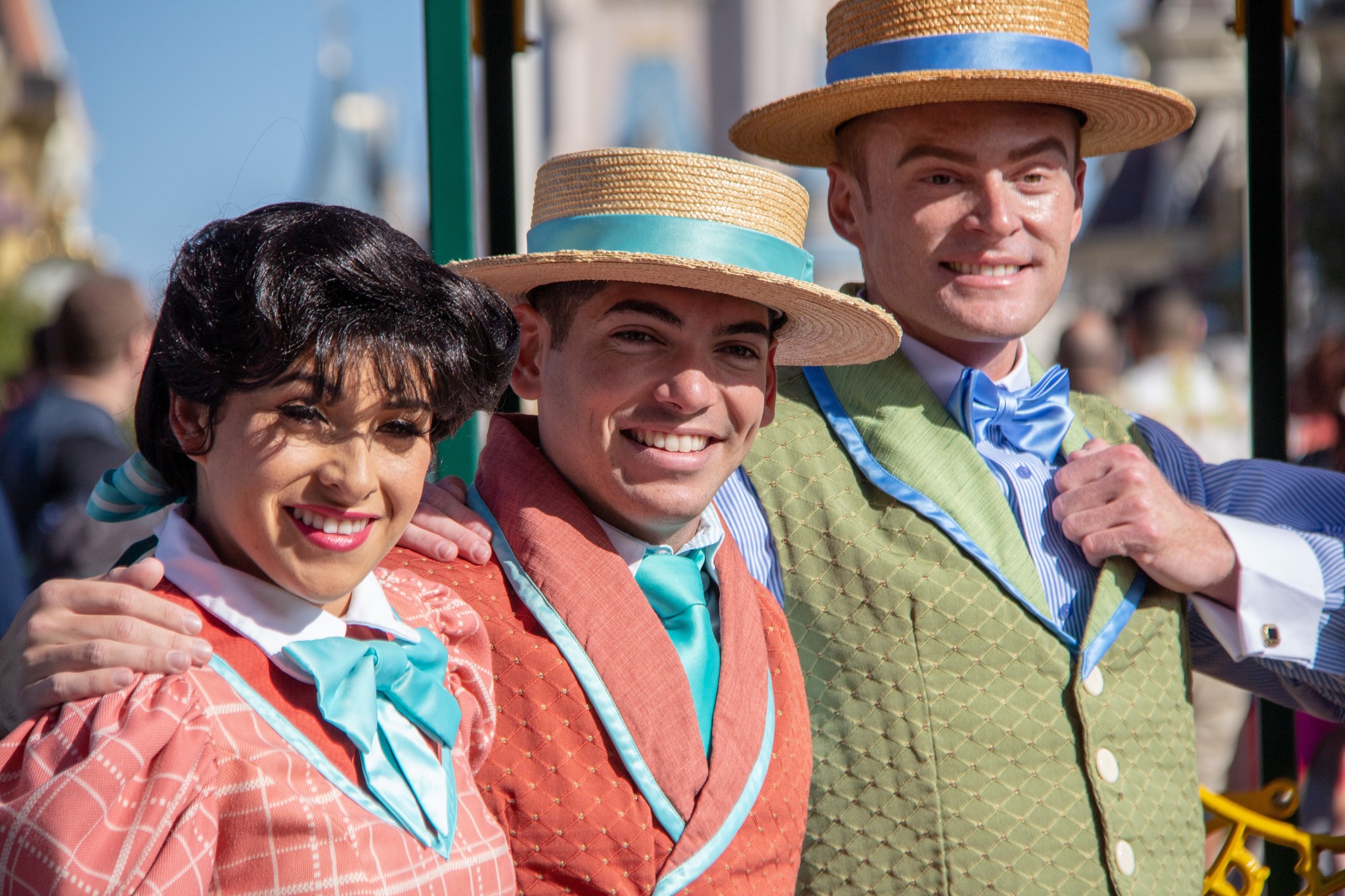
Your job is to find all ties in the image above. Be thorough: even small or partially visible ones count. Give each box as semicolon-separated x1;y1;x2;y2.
634;547;720;762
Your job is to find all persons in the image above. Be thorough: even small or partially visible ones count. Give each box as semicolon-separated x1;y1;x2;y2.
0;203;522;896
0;147;904;896
0;281;169;595
399;1;1345;896
1058;282;1345;895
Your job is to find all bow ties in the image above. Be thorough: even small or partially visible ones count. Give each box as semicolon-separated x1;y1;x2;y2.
944;363;1074;465
281;609;463;846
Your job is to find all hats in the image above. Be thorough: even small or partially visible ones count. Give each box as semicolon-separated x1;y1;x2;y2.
728;0;1198;167
441;147;903;365
